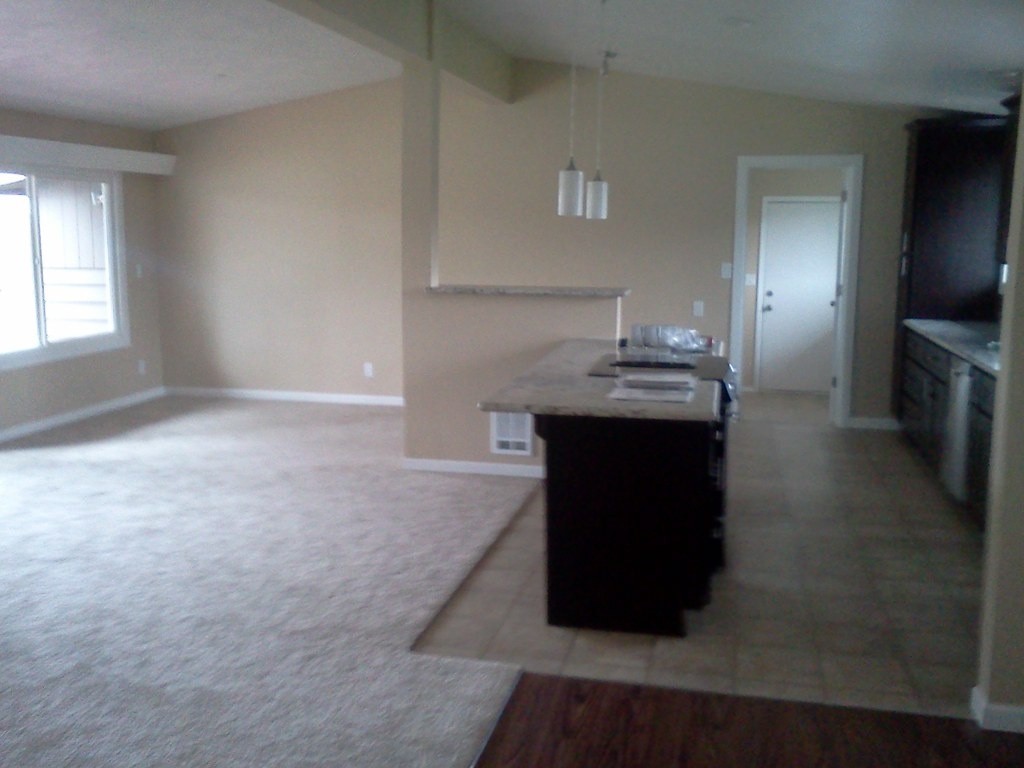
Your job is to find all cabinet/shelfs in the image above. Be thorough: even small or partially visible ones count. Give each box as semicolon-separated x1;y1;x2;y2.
894;327;996;505
532;416;729;640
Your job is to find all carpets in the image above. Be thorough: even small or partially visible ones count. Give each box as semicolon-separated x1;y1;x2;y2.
0;442;523;768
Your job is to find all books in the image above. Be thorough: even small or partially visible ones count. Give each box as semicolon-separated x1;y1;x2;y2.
610;372;699;403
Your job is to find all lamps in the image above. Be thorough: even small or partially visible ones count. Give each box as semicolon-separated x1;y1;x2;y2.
558;43;585;217
585;50;619;219
